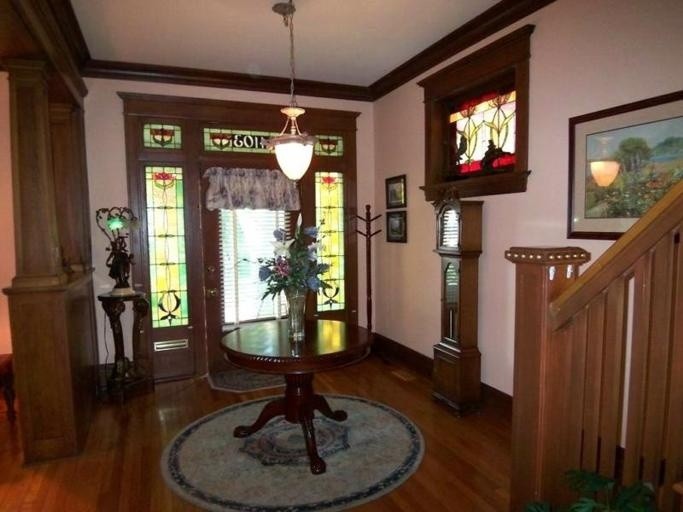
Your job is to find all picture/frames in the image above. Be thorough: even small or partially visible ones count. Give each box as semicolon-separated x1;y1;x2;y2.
386;174;407;243
568;90;683;244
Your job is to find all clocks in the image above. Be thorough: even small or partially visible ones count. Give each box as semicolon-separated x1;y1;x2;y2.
432;185;484;416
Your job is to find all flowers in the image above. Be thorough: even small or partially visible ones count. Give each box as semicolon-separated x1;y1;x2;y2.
244;219;339;299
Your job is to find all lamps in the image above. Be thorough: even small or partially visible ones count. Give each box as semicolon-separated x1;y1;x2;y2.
587;136;621;187
266;0;319;180
96;206;140;295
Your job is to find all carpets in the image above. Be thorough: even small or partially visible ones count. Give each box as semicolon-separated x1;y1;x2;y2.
161;393;425;512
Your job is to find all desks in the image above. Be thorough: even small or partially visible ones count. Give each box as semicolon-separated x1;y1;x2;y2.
98;290;154;405
221;318;374;474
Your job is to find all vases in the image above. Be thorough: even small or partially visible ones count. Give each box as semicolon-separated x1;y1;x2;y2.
283;289;308;343
